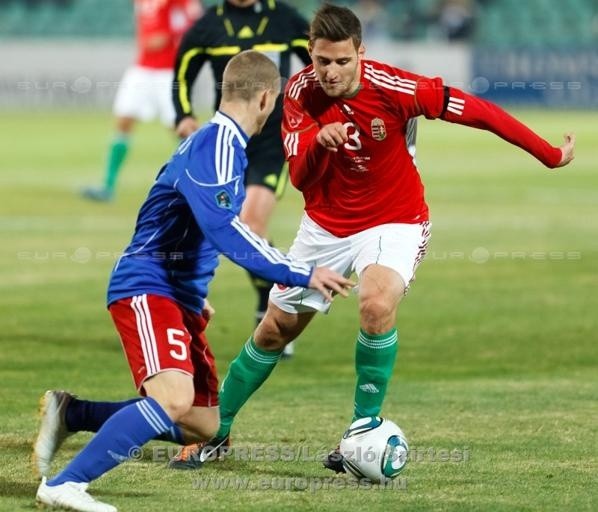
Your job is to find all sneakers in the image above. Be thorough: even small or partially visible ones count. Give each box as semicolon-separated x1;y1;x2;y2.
36;474;118;512
168;432;231;470
31;390;79;480
323;446;348;474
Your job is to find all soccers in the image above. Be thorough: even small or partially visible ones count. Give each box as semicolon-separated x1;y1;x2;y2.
339;416;410;485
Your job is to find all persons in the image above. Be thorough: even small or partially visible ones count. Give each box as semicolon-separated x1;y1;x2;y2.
170;3;577;469
393;2;478;44
77;0;216;204
349;1;395;43
169;1;316;359
33;49;360;512
